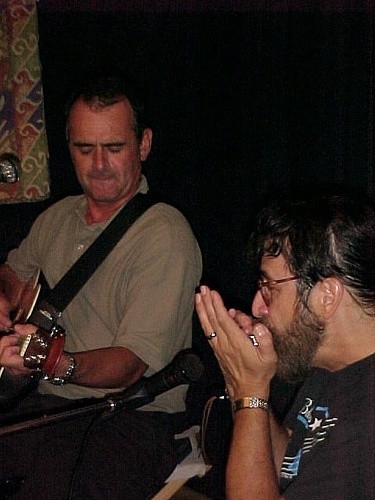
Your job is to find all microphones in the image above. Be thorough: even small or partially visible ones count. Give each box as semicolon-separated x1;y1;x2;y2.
90;348;205;428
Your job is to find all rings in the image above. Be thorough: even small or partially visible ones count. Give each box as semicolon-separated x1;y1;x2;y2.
203;330;217;341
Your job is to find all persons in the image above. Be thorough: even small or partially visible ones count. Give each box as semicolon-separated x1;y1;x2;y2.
0;77;202;500
195;192;374;500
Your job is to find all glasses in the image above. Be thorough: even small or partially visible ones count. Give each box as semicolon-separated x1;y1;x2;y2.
257;273;310;309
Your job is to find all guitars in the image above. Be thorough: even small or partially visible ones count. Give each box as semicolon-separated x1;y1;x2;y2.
0;267;61;403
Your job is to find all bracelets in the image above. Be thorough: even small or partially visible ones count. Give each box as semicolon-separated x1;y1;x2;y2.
230;397;270;417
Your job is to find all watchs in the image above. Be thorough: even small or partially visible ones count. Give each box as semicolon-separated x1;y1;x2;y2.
40;349;77;386
